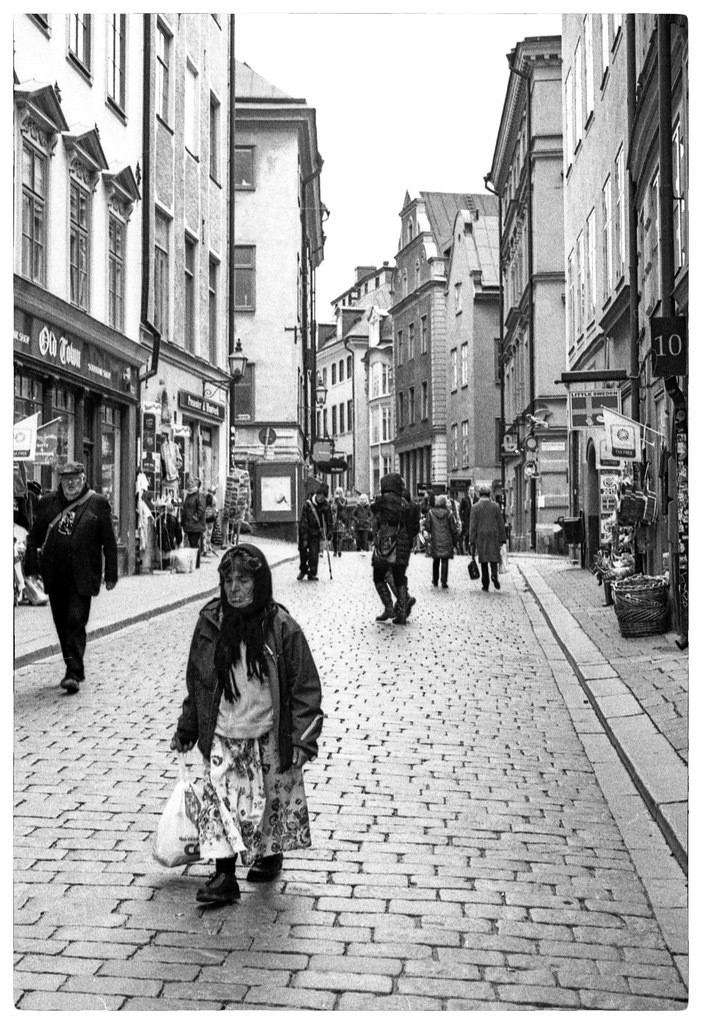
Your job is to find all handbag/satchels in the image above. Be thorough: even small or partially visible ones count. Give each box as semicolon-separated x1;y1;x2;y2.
152;751;202;868
370;523;398;568
422;528;437;557
499;544;510;574
468;556;480;580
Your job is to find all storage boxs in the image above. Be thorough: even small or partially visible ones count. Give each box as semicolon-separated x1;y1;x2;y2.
168;548;200;574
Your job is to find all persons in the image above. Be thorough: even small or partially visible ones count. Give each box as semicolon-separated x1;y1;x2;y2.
297;473;507;625
169;544;324;903
181;478;216;568
22;463;118;693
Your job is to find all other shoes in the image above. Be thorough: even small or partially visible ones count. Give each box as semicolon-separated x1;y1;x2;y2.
60;672;85;695
442;583;449;588
196;870;241;902
308;576;319;580
246;852;284;882
481;585;488;592
491;574;501;589
297;571;305;580
431;580;438;586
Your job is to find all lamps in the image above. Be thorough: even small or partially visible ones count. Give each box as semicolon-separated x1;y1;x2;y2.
202;338;249;398
297;380;328;410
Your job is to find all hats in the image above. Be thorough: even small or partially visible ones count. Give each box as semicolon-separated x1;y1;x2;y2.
57;461;84;475
316;483;329;498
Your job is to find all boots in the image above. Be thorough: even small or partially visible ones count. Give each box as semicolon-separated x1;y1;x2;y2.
376;576;398;623
392;584;408;625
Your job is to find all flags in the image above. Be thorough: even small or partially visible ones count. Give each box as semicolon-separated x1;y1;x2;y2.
591;407;641;471
